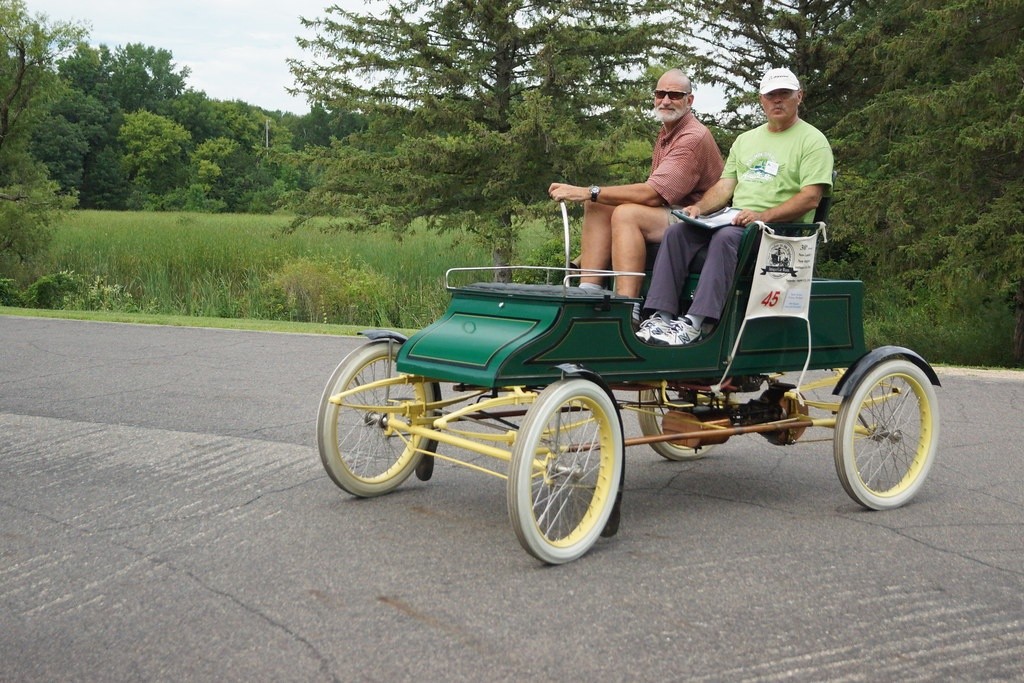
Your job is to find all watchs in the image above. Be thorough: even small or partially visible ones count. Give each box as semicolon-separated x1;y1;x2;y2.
589;184;601;203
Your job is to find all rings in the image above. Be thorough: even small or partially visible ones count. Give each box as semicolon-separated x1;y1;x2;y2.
744;219;748;222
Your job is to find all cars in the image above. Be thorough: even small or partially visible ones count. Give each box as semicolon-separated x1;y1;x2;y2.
316;173;942;564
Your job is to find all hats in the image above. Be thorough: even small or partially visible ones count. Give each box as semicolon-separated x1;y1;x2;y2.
759;67;801;96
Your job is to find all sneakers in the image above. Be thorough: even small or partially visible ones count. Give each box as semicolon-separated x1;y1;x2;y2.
635;313;670;342
649;315;702;348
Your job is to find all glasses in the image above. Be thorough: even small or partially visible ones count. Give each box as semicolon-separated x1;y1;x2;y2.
762;88;795;100
653;90;691;100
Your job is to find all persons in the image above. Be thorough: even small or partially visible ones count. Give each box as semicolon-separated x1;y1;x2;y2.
548;68;732;332
634;68;835;348
